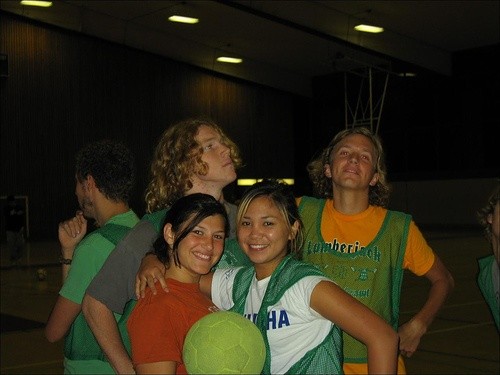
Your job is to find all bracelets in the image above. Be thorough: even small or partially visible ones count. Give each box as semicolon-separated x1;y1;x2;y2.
62;258;72;264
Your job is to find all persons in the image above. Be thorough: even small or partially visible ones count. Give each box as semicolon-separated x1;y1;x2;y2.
135;182;399;375
477;189;500;329
82;117;238;375
295;126;455;375
45;140;140;375
128;193;231;375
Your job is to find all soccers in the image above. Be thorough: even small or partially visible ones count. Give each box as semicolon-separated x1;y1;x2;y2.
181;310;266;375
